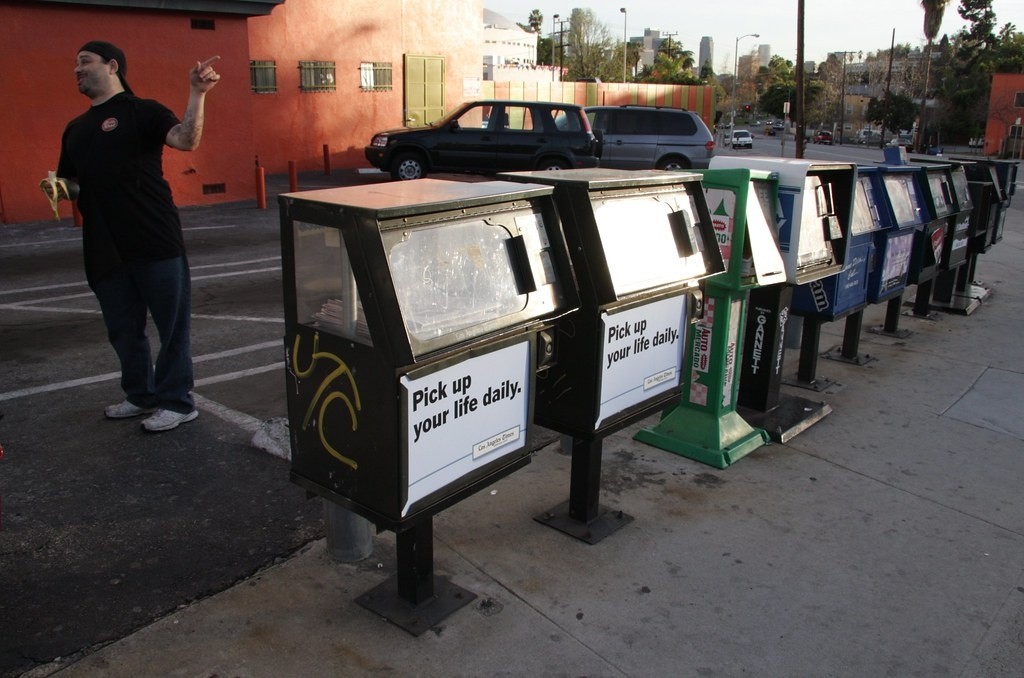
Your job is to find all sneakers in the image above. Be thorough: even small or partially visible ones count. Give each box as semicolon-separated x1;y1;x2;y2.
103;398;157;419
140;407;200;433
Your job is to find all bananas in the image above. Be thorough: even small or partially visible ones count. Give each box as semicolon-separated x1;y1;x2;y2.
40;170;70;218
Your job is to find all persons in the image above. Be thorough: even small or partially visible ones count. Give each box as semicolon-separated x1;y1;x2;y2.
39;39;223;432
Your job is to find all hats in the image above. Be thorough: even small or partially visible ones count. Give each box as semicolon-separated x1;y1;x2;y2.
77;40;132;91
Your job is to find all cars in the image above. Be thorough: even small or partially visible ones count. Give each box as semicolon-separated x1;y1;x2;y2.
766;119;774;125
969;134;984;148
856;129;944;156
813;130;833;145
767;120;786;136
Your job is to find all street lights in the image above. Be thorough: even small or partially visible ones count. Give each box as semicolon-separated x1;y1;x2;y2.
552;14;560;81
620;8;627;82
730;34;760;148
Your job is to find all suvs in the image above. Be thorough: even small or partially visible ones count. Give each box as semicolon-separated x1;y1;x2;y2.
556;101;715;172
364;100;601;181
732;129;754;149
